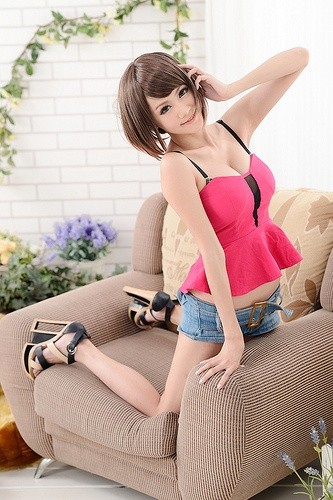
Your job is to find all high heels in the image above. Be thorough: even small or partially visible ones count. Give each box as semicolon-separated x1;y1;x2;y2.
122;286;176;330
21;318;91;381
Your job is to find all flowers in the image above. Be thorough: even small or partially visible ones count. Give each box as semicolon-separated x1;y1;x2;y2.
44;213;117;261
277;416;333;500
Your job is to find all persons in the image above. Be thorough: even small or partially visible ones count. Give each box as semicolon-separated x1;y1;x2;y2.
21;47;309;418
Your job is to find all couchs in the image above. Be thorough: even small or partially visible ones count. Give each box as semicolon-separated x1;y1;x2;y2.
0;187;333;500
